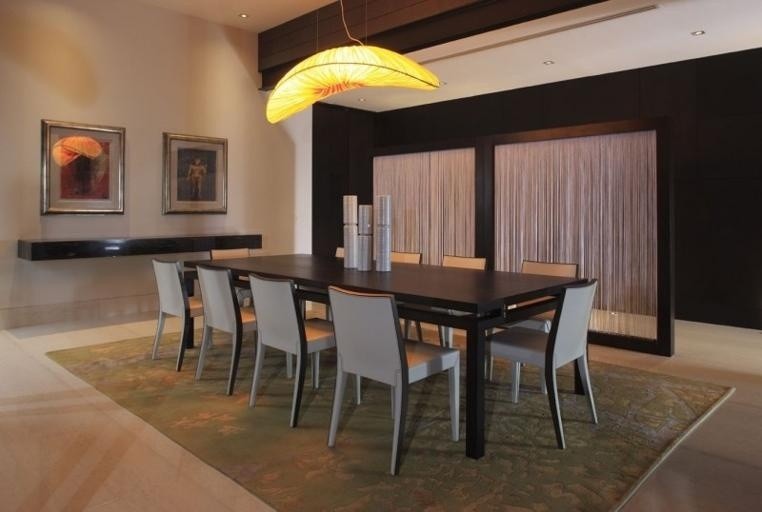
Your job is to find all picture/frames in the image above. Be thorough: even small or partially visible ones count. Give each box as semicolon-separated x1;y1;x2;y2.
164;132;227;212
42;120;124;214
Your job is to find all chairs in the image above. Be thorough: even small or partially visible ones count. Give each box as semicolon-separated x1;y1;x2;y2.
442;255;487;270
194;263;256;396
481;278;602;452
249;273;362;427
389;251;422;264
209;248;250;261
328;286;462;477
522;258;578;322
151;258;202;371
336;247;345;259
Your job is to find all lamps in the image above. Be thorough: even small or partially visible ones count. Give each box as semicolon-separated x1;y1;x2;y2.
264;0;440;123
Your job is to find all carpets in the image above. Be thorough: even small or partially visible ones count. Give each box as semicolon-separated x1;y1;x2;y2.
47;326;736;512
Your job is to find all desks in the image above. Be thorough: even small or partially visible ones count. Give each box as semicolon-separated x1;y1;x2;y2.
185;255;586;457
